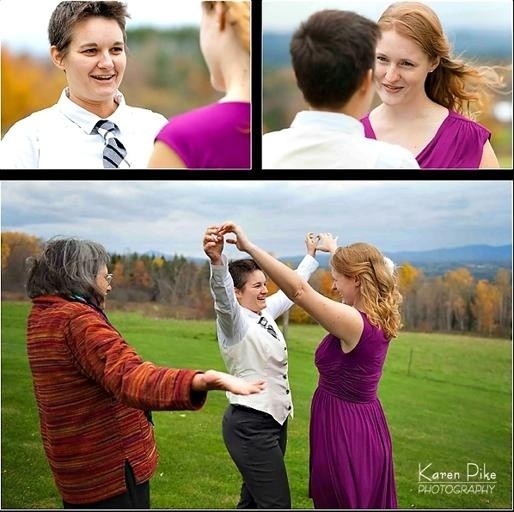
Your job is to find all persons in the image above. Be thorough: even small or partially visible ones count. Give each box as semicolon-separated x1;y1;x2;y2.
358;0;513;172
261;8;420;169
0;1;172;171
202;223;322;509
218;219;405;509
24;231;267;510
148;0;251;171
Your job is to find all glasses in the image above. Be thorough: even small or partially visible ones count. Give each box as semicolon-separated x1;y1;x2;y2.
93;273;113;284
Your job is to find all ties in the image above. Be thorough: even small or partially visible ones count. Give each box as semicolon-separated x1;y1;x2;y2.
259;317;279;340
95;120;131;170
